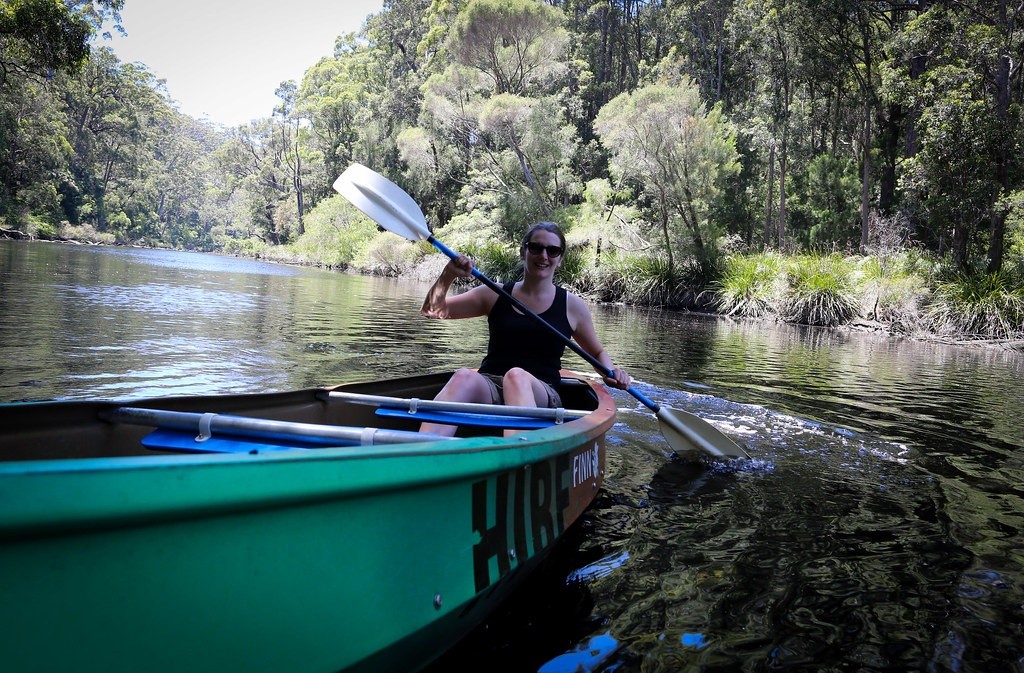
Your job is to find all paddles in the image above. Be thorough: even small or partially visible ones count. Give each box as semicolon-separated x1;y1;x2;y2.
330;161;754;464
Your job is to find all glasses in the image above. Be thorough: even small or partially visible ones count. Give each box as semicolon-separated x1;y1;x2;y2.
524;242;564;258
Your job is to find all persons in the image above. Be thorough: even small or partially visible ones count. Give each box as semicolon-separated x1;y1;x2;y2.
418;221;630;438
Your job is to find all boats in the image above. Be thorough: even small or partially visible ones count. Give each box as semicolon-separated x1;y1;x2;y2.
1;365;617;672
